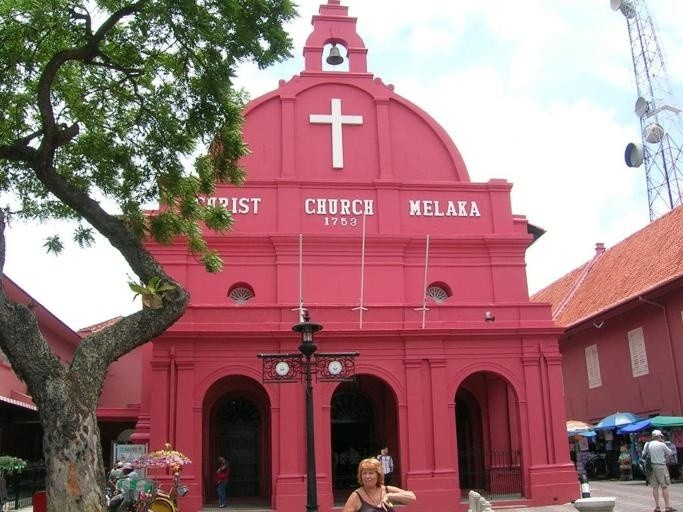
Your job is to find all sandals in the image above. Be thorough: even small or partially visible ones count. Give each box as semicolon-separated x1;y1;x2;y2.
664;507;677;512
653;507;661;512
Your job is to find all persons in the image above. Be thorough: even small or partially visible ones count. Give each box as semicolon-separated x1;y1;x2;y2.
641;429;678;511
663;440;679;483
376;445;393;485
105;460;138;511
340;458;417;512
215;455;230;508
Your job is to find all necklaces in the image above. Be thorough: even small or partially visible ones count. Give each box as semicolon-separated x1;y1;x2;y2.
362;485;384;507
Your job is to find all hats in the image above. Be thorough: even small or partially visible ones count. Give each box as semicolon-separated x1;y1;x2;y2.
652;429;665;437
119;463;134;470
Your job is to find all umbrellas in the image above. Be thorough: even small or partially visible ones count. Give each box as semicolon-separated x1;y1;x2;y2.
131;449;192;469
566;411;683;443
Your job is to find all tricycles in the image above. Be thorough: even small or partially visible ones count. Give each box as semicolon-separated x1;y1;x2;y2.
105;450;191;512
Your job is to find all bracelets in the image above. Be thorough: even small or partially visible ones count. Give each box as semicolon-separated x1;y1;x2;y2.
387;492;392;501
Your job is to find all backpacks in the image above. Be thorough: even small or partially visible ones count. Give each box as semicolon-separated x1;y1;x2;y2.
639;441;653;476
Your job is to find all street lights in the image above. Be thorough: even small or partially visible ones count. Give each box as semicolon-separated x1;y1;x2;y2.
292;310;323;512
